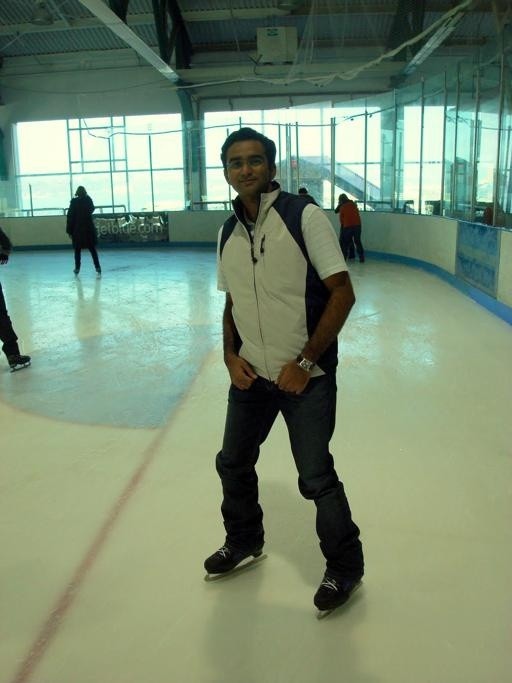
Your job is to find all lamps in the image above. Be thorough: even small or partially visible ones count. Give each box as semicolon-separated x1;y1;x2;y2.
30;0;53;26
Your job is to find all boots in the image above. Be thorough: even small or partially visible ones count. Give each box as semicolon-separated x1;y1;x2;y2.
2;342;31;368
314;567;364;609
204;536;264;573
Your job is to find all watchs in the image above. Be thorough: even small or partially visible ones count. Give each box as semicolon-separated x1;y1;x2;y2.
296;354;315;372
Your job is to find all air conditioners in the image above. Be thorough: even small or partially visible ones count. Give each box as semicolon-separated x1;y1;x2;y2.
256;26;298;64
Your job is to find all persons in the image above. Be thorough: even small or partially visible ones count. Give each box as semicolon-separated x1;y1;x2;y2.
203;127;366;611
1;226;31;370
297;188;317;205
334;193;366;262
65;185;101;275
337;193;357;259
